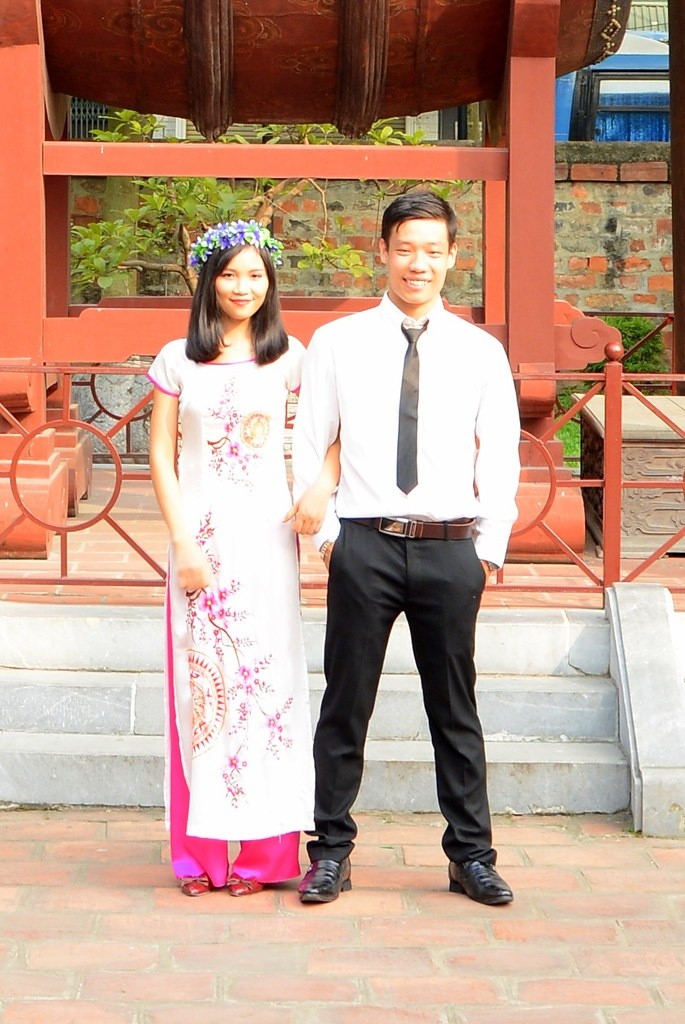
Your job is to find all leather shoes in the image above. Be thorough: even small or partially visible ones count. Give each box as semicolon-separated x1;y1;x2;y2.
298;856;352;903
227;873;266;897
448;859;514;903
177;875;212;896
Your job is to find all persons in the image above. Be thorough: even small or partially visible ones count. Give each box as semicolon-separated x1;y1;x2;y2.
149;220;342;898
291;191;525;907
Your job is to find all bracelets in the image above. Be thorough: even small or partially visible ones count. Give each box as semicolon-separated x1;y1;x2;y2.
319;540;332;561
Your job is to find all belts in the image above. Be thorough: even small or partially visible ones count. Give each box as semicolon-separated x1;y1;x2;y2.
350;517;477;540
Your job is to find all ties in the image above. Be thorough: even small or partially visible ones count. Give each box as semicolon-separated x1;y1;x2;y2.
396;317;430;495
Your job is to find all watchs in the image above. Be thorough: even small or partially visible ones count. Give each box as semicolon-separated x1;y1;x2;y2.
486;561;499;573
